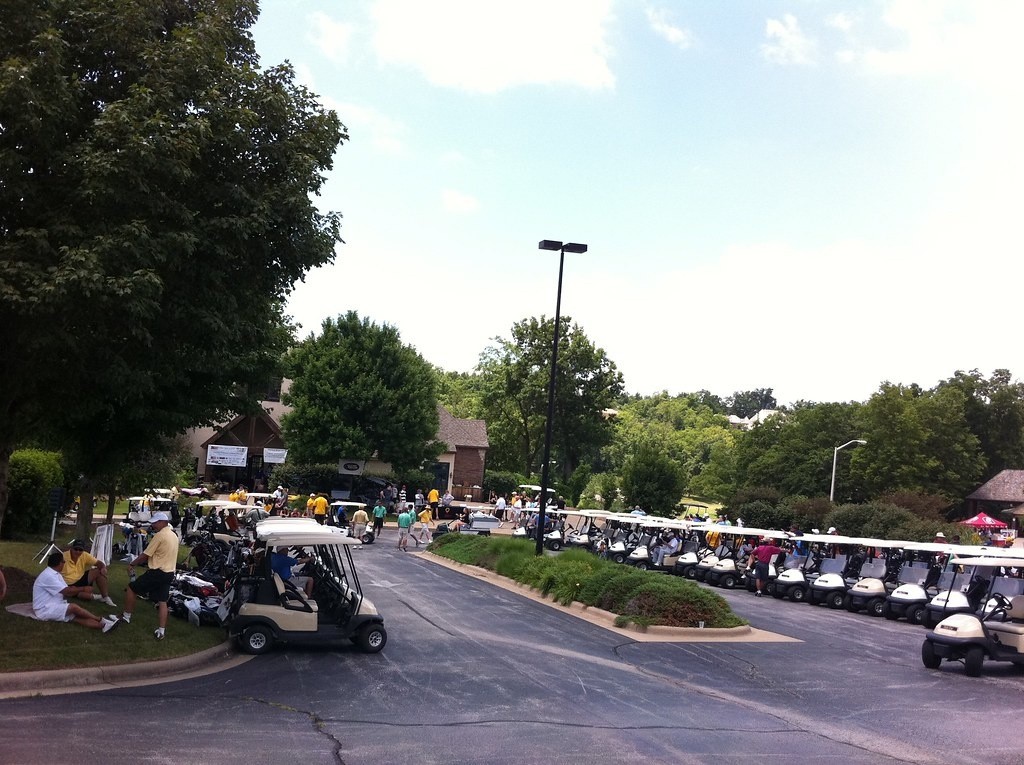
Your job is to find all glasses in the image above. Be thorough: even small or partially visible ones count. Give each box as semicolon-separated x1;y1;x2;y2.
403;487;406;489
61;561;65;563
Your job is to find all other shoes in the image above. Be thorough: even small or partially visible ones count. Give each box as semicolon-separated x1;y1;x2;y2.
508;519;514;522
450;528;458;532
337;523;346;529
373;533;380;539
499;522;505;528
501;520;504;522
754;591;762;597
741;574;746;578
529;538;534;541
655;562;661;566
398;547;407;552
436;517;440;520
396;514;399;517
353;545;363;550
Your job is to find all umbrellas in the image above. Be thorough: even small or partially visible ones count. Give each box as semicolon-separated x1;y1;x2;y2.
959;512;1007;531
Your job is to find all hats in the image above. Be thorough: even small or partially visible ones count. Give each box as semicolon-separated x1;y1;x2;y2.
276;545;293;553
635;505;640;509
812;528;819;534
310;493;315;497
788;524;798;529
425;505;432;510
72;538;84;550
702;513;709;518
278;486;283;489
826;526;836;533
761;537;774;543
666;532;674;537
148;511;169;524
935;532;946;538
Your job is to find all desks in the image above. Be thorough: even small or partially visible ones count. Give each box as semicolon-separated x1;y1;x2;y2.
485;507;512;521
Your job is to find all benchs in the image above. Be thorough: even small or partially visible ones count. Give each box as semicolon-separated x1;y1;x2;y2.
582;523;1023;635
126;511;151;526
333;516;352;530
271;569;318;613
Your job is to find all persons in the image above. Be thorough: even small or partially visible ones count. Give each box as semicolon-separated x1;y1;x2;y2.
489;491;566;541
452;508;470;532
33;540;121;635
272;546;316;600
337;484;454;552
952;535;965;574
930;532;949;572
226;466;331;538
109;513;179;640
137;482;182;528
630;506;731;567
199;484;211;500
738;525;840;597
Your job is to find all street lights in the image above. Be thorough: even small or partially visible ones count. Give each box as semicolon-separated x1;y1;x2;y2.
829;440;867;502
535;239;589;558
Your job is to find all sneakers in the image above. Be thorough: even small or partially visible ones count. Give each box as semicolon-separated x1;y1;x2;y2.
108;611;131;625
418;538;434;543
93;594;118;607
152;627;166;641
101;617;122;636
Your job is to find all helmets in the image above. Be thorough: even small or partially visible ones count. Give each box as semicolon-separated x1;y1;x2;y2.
718;515;726;521
